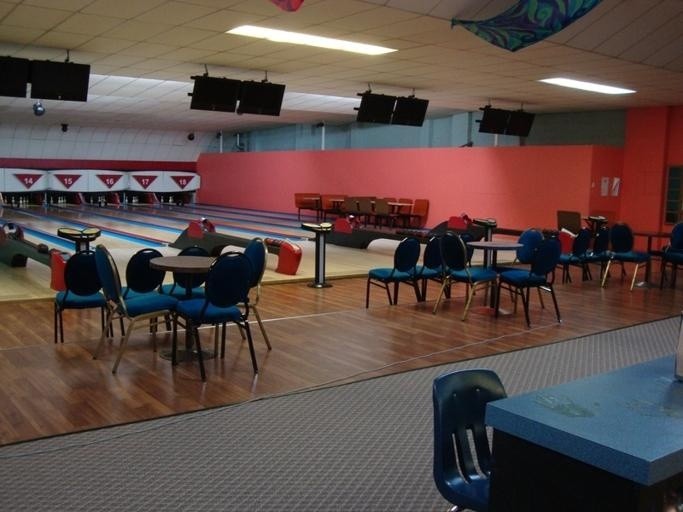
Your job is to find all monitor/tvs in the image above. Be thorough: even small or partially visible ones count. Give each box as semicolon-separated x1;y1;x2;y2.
357;93;396;124
506;111;535;136
191;75;241;112
478;108;511;135
391;97;429;126
31;59;90;102
237;80;285;116
0;55;29;97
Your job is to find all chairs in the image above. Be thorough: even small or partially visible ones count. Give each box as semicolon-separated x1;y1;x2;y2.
427;365;522;511
38;236;277;380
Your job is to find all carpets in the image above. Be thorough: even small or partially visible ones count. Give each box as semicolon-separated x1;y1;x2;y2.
0;305;681;511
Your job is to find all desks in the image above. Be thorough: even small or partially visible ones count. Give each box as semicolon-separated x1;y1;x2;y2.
482;350;682;512
